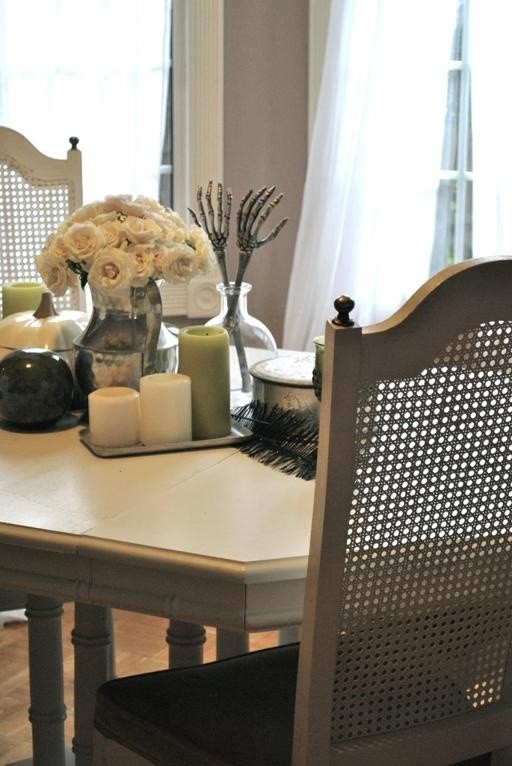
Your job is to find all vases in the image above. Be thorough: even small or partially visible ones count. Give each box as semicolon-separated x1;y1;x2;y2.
72;276;179;425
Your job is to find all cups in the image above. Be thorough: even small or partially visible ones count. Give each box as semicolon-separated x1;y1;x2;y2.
311;336;379;471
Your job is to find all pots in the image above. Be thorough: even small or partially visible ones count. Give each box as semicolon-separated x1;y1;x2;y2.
251;352;320;431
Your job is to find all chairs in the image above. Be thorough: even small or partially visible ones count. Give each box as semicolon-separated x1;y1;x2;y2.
0;126;82;318
92;254;512;766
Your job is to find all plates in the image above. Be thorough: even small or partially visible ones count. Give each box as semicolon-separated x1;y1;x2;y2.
78;417;255;459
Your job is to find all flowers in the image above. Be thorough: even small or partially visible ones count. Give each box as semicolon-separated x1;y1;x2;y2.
34;193;216;296
